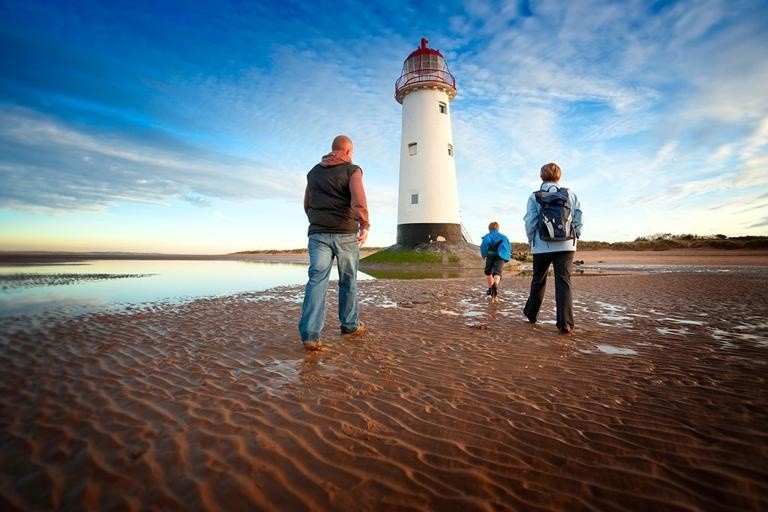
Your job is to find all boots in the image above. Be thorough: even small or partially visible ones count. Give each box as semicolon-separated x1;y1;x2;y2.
487;283;498;297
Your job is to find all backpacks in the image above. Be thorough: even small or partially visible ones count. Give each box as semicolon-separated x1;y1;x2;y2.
533;187;576;246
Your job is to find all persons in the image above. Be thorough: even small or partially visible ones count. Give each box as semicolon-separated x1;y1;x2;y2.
299;135;370;352
524;162;584;333
480;222;512;299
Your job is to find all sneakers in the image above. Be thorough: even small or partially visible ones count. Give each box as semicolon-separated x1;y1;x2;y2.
342;321;366;337
304;341;322;351
